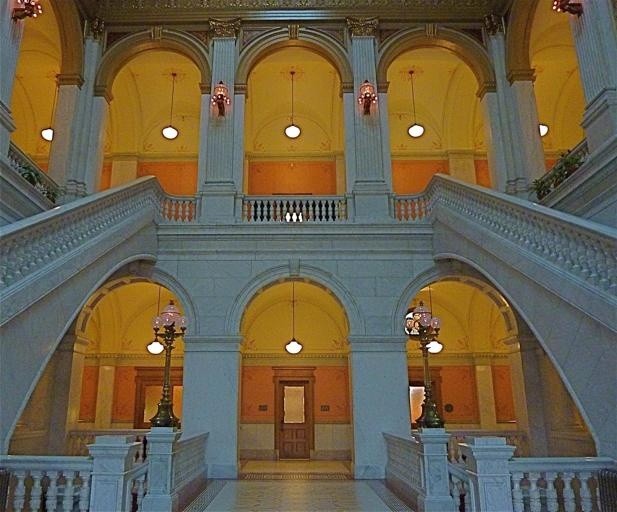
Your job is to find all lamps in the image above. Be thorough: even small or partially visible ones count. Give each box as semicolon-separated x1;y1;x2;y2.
212;80;230;118
402;298;446;432
149;298;188;428
356;79;380;115
